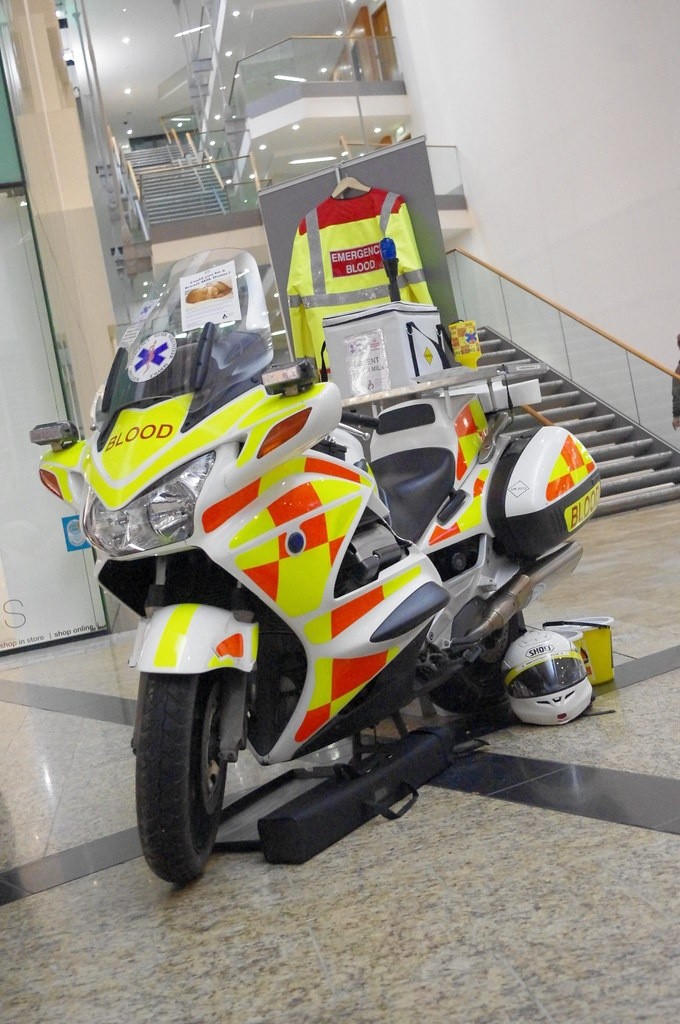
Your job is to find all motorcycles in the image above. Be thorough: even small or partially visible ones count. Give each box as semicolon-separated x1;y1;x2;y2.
31;243;606;887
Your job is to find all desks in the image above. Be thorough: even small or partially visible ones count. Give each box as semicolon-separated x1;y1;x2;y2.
341;362;549;419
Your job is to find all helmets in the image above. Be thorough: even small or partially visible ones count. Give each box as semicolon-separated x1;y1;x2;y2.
502;630;595;725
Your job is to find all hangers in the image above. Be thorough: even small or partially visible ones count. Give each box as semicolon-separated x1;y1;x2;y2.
332;159;371;198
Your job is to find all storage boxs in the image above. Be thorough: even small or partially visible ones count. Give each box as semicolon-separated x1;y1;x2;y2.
440;378;543;419
322;300;448;398
